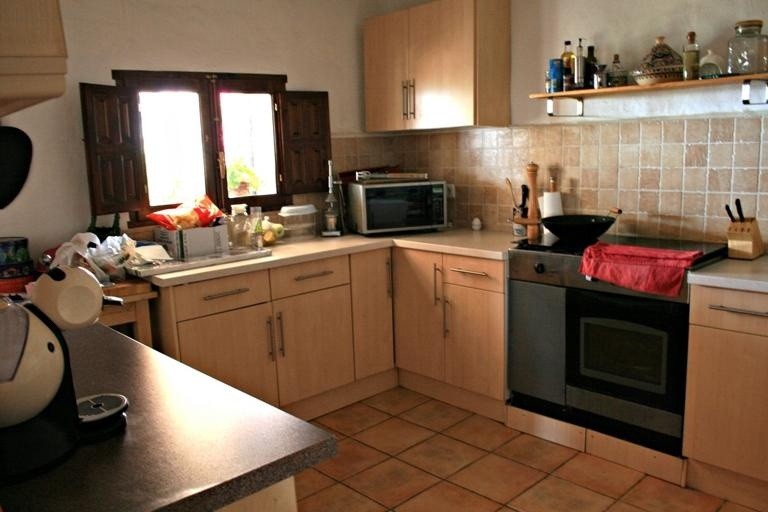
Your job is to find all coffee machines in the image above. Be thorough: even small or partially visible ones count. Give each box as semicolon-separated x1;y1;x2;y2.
1;262;133;485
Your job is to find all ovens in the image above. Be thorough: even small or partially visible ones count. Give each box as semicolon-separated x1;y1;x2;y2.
560;285;689;456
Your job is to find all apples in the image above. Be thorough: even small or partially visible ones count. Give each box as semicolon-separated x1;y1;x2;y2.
272;223;285;239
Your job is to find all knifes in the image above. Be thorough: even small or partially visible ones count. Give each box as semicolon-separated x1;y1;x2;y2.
724;198;746;223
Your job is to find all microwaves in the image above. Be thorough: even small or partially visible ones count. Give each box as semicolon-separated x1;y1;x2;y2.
344;173;448;237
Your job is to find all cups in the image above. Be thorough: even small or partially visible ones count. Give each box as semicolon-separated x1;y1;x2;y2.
511;206;528;237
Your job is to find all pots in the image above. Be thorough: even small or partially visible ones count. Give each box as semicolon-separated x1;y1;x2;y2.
532;205;622;245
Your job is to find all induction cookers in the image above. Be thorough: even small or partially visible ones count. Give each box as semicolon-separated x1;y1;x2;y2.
503;231;729;274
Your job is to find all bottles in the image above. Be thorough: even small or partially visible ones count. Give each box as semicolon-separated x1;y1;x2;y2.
543;18;768;94
225;201;266;251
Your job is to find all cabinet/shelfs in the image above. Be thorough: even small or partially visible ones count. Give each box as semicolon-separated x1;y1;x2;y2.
360;0;513;132
167;249;354;423
682;270;768;512
393;240;509;424
350;248;395;382
